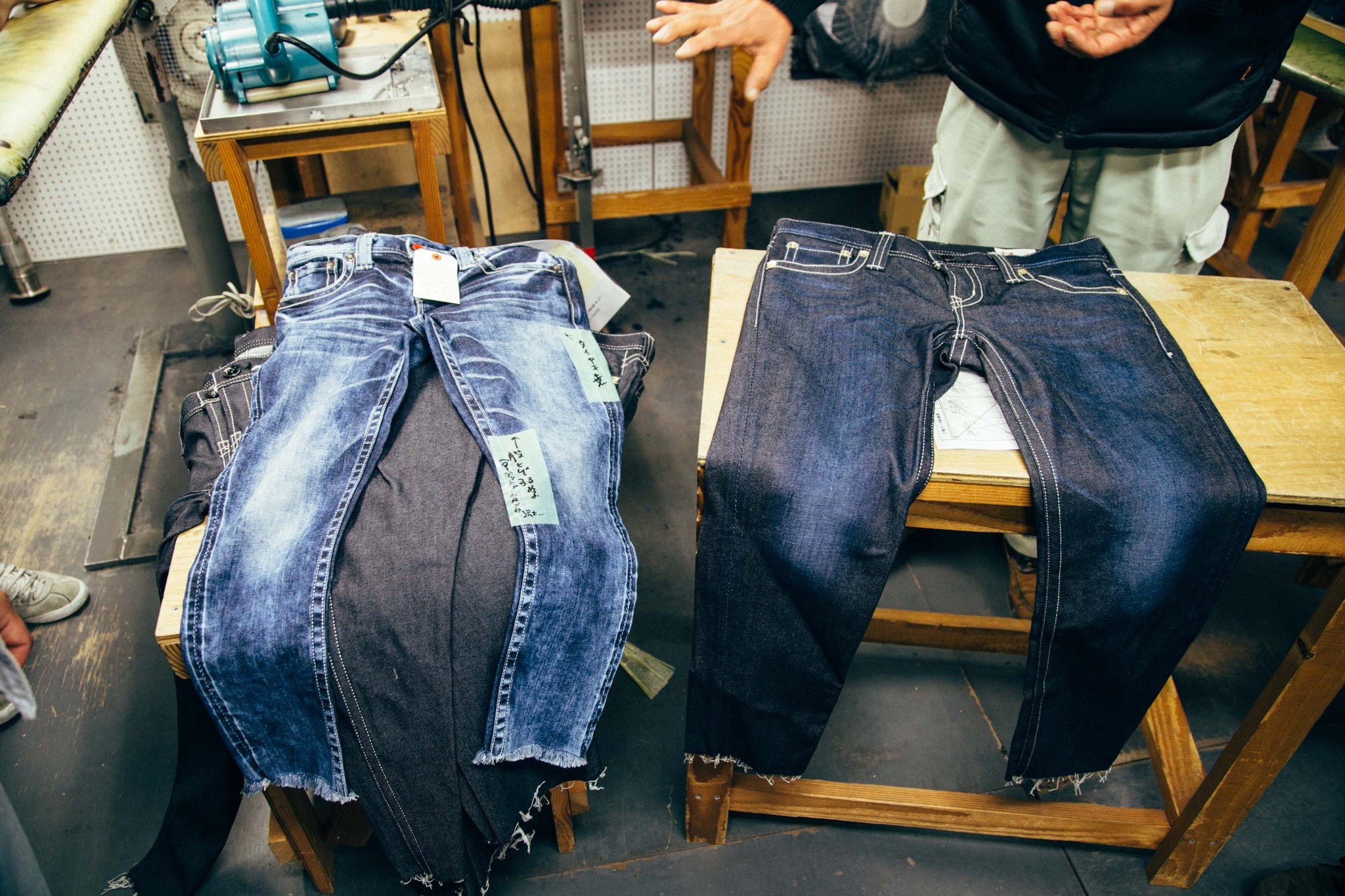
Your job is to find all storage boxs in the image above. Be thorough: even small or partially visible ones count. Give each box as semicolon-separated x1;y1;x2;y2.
879;162;932;238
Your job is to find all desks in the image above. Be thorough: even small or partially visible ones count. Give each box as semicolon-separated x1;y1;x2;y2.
687;248;1345;890
1203;10;1345;303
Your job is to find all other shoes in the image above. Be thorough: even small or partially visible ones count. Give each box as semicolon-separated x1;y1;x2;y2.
1007;545;1038;622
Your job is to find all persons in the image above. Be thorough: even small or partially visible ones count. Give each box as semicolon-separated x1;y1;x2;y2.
0;561;90;724
644;0;1310;620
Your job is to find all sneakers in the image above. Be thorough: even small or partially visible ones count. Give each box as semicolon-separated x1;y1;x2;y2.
0;692;20;724
0;564;91;623
